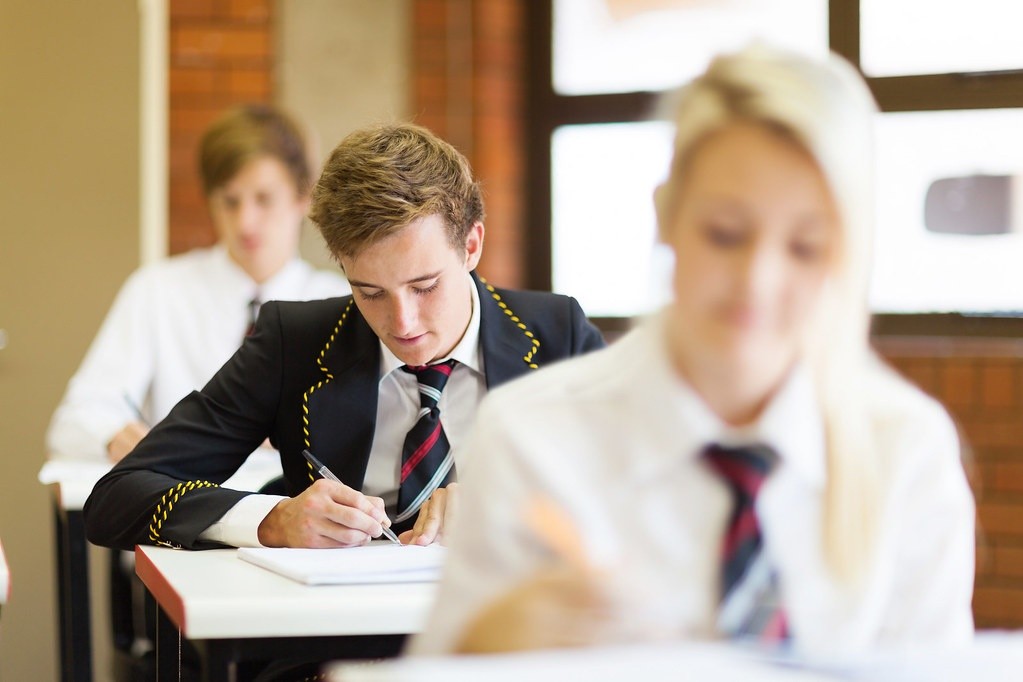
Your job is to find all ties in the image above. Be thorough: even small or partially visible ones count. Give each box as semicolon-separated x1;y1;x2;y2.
244;297;262;335
704;441;788;639
384;358;458;540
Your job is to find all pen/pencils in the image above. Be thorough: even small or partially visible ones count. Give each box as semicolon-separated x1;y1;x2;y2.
519;490;605;585
124;392;153;426
301;447;405;548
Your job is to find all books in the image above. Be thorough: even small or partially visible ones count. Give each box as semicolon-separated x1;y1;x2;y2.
235;544;444;585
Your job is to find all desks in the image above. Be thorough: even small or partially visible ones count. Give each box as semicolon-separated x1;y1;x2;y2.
48;457;1022;682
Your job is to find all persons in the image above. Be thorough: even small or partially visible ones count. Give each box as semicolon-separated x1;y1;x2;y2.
407;44;975;655
84;121;607;682
48;104;354;681
